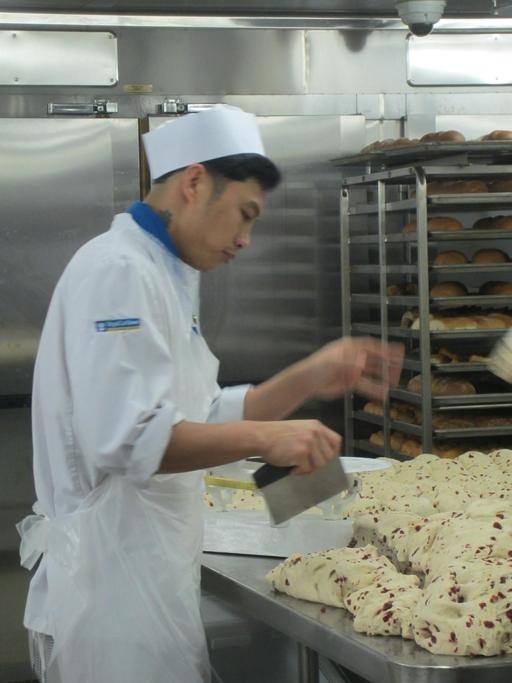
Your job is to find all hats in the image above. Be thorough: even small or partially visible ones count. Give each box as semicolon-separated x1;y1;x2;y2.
141;107;265;179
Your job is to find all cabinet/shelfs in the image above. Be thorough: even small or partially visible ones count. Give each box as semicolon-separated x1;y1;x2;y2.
337;161;512;460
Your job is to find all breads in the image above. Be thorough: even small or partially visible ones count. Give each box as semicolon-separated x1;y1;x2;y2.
360;131;512;459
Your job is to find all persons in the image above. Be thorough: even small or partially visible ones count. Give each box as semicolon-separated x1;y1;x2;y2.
16;100;412;682
